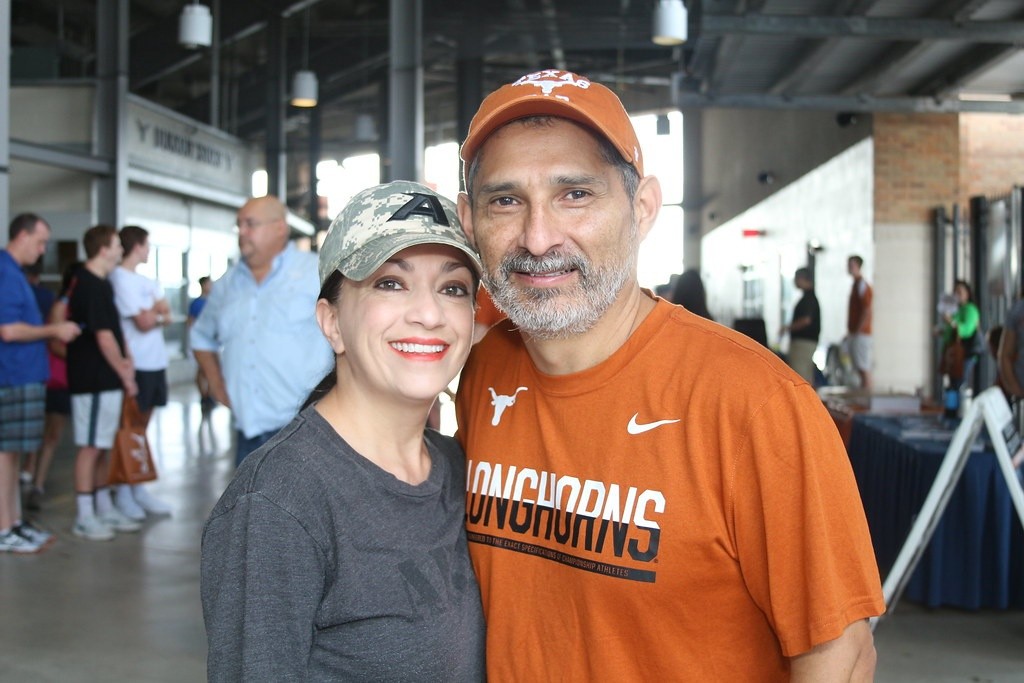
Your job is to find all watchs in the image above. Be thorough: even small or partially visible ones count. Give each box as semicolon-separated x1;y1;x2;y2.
156;313;164;325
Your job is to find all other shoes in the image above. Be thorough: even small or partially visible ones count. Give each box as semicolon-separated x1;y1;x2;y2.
14;521;56;543
0;531;41;552
113;502;146;519
98;510;141;531
137;498;170;513
27;490;42;508
73;522;115;539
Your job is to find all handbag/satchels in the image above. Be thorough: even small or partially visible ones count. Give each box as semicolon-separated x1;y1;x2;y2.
941;331;964;379
108;398;158;483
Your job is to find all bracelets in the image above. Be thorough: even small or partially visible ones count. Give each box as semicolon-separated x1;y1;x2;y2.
950;320;955;327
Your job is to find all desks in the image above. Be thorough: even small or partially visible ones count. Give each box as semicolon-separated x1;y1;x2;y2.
852;411;1024;611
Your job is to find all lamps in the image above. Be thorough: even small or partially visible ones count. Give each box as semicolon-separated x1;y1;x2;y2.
650;0;688;44
179;0;213;49
290;6;318;107
656;114;671;135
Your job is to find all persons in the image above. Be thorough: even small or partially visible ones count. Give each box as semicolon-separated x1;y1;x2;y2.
198;179;485;683
941;281;986;429
456;70;887;683
188;275;218;417
846;258;873;389
186;193;335;467
0;213;171;554
998;301;1023;428
783;267;820;382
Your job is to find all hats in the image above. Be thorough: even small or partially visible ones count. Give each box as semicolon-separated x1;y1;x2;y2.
459;69;644;195
318;180;483;297
